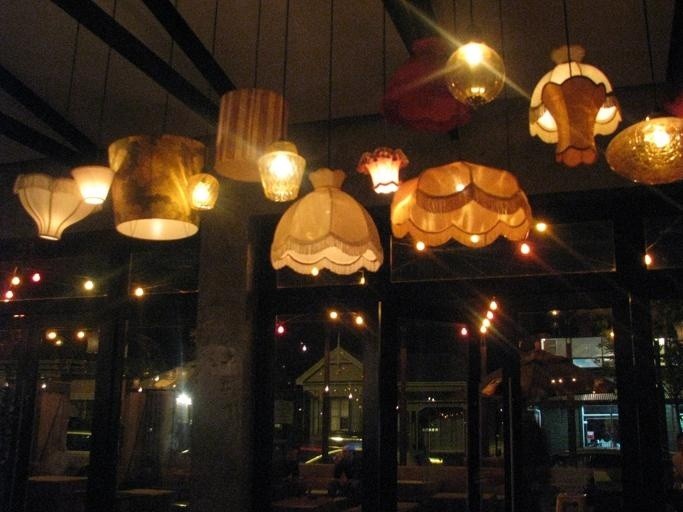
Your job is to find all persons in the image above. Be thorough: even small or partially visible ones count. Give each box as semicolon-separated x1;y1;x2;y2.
324;441;363;509
670;432;683;483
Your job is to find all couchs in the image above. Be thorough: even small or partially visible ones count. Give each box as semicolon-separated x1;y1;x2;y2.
272;463;505;510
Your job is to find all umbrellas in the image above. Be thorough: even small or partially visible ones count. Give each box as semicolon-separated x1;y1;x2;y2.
478;341;618;402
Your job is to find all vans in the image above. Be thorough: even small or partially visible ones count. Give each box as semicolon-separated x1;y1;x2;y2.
59;429;93;477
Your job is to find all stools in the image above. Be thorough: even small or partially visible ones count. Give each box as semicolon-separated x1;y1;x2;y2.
555;492;587;512
